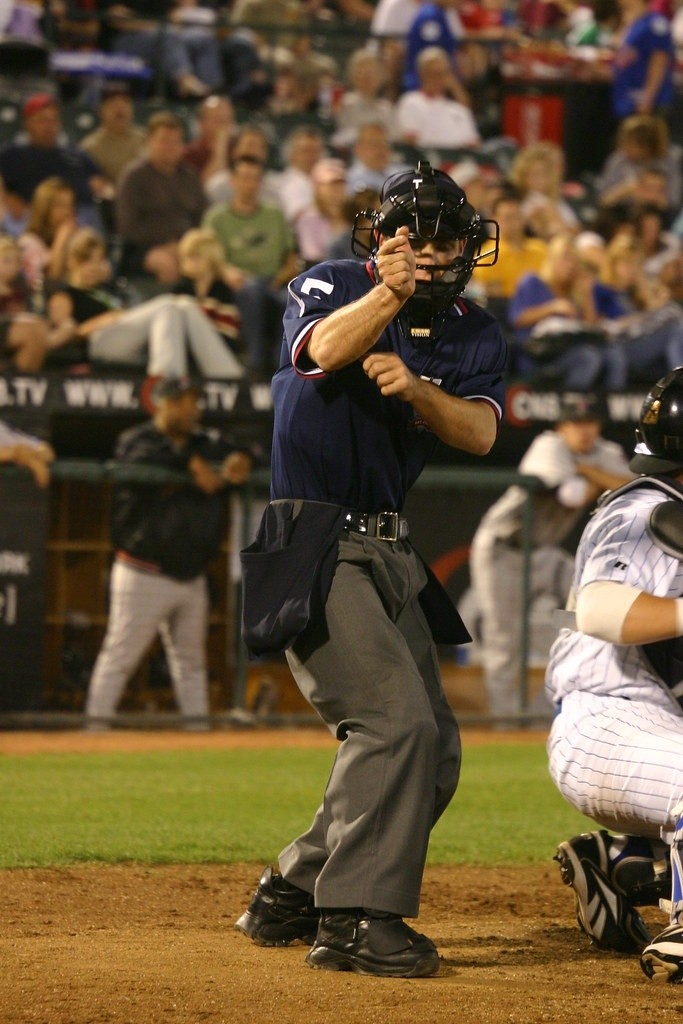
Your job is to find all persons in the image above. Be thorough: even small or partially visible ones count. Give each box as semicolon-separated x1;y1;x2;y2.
87;369;250;734
233;159;509;977
545;364;683;984
0;0;683;488
468;387;636;733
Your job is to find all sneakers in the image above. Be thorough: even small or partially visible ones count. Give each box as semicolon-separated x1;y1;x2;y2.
305;908;440;978
640;920;683;985
232;867;318;947
552;825;651;957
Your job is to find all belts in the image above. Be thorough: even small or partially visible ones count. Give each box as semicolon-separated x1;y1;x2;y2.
344;509;412;542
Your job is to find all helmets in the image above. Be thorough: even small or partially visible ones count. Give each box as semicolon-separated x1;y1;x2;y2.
629;366;683;473
351;161;500;341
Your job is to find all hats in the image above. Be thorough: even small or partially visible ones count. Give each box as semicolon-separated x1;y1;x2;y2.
150;375;210;403
552;392;604;424
311;158;351;184
